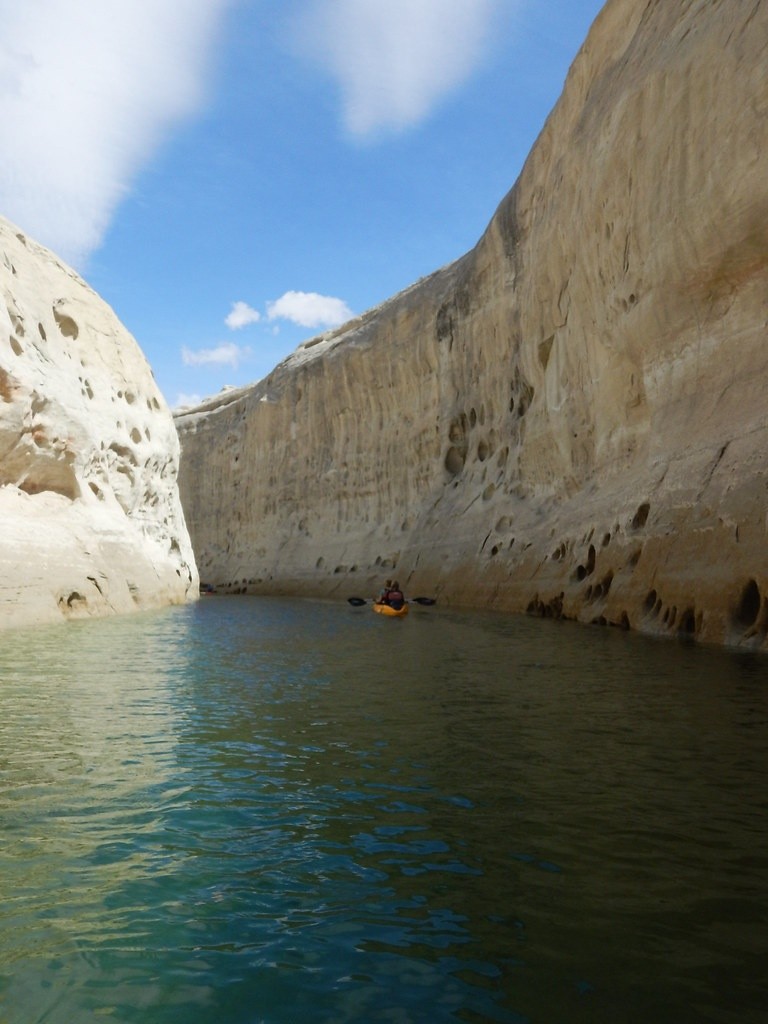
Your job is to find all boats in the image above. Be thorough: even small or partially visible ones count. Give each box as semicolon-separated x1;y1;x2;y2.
372;602;411;617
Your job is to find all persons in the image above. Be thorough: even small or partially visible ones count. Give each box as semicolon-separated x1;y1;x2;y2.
378;579;403;610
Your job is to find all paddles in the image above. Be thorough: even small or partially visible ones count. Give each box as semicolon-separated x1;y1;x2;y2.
347;597;437;607
